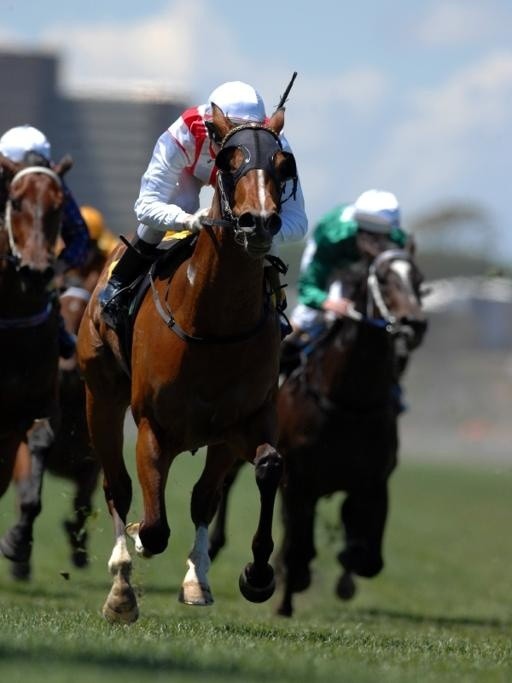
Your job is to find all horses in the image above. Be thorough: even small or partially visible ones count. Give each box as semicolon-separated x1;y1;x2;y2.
0;148;74;496
76;100;296;626
1;236;111;581
272;233;428;620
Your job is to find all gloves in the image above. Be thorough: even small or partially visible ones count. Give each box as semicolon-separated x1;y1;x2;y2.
173;209;210;232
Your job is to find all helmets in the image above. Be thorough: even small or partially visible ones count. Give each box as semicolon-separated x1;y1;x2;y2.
0;125;52;166
208;79;267;125
354;188;401;236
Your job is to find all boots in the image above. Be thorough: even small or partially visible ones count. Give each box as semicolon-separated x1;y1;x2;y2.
97;233;157;319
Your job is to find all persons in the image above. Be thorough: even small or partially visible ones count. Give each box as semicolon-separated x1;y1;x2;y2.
43;203;125;338
99;79;310;389
276;186;431;417
1;122;94;363
